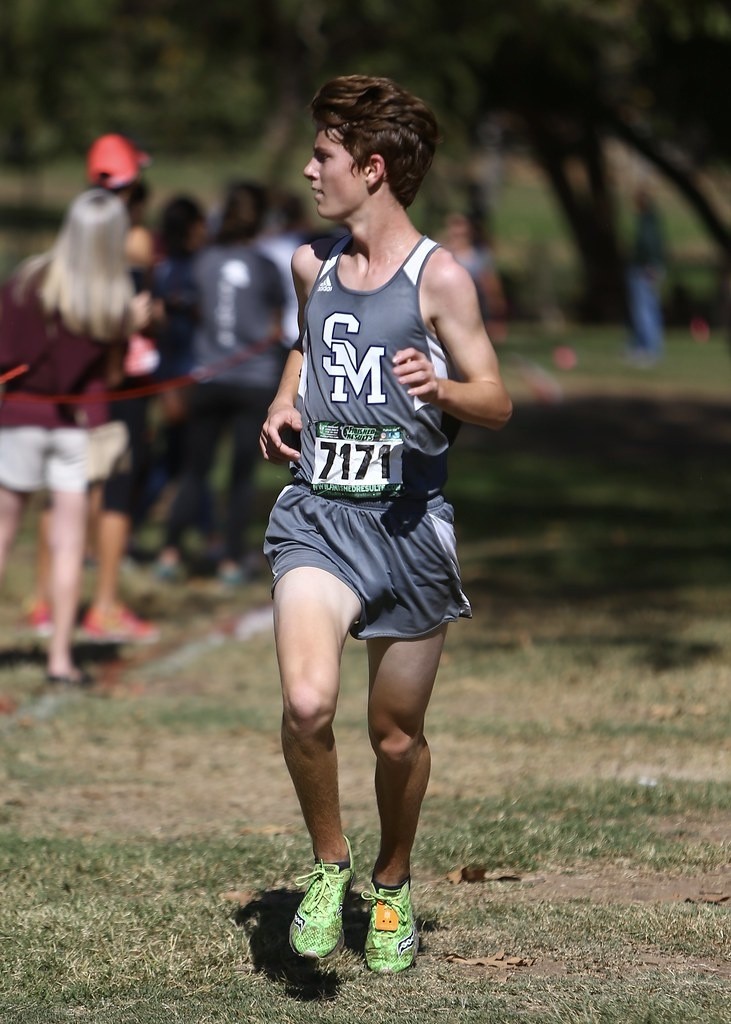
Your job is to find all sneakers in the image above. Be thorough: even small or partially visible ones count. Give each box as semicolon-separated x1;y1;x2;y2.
29;600;56;639
288;834;357;962
84;609;163;645
362;880;420;974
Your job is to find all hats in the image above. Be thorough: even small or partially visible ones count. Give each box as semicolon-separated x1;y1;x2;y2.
87;134;148;187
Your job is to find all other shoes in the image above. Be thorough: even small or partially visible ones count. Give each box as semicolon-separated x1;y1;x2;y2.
47;662;92;688
218;549;245;586
151;538;188;584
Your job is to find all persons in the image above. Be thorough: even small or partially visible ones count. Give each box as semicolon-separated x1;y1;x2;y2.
259;74;512;974
113;113;686;585
19;134;164;641
2;188;154;688
